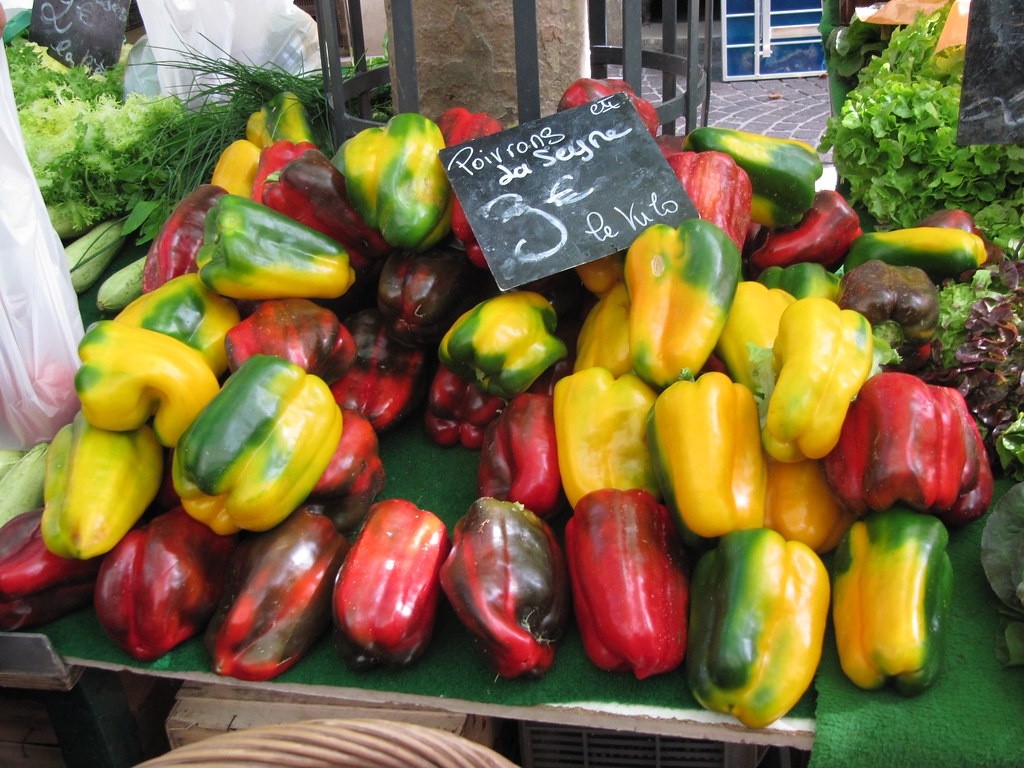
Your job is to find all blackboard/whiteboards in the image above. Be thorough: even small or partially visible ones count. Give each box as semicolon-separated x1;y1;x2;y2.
955;0;1024;147
28;0;132;77
438;91;701;291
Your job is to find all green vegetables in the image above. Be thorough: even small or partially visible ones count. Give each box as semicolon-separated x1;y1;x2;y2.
2;40;219;246
817;0;1023;667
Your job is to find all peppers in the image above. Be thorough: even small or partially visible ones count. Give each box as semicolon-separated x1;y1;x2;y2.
0;78;997;732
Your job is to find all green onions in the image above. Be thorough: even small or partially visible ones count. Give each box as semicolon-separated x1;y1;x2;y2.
70;23;394;274
